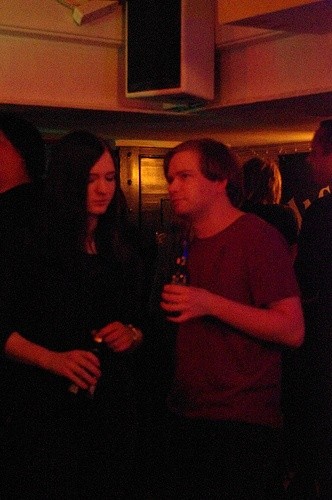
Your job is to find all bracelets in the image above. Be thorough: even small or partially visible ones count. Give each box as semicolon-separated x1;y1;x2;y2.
124;324;142;348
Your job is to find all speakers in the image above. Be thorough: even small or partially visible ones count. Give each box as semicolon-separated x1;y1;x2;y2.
124;0;216;102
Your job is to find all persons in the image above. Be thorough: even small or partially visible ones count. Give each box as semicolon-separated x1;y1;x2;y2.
297;118;332;500
160;136;305;497
0;129;158;500
238;157;300;241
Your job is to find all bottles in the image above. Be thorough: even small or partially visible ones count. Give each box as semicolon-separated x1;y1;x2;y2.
85;329;107;399
170;240;190;286
